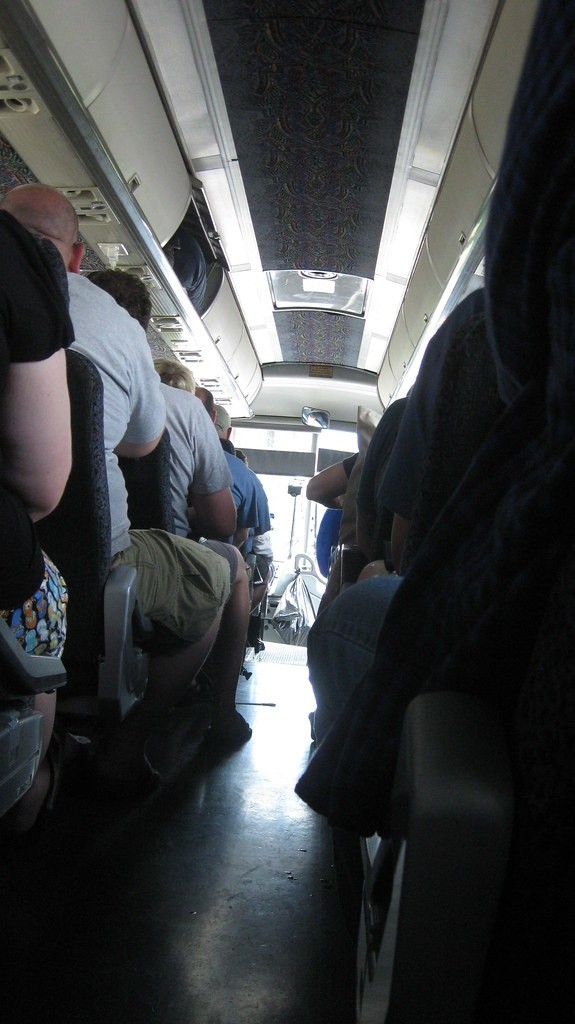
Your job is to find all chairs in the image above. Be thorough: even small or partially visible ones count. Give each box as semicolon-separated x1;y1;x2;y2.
117;427;177;535
353;549;575;1024
34;345;152;728
241;536;257;600
0;615;67;817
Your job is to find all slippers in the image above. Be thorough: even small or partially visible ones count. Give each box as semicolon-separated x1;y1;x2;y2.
103;755;160;797
43;732;61;811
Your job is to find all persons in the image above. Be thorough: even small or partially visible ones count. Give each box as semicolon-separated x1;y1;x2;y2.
0;182;271;898
304;413;322;427
295;0;575;1024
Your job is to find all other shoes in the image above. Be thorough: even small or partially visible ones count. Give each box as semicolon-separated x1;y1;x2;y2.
213;710;251;745
